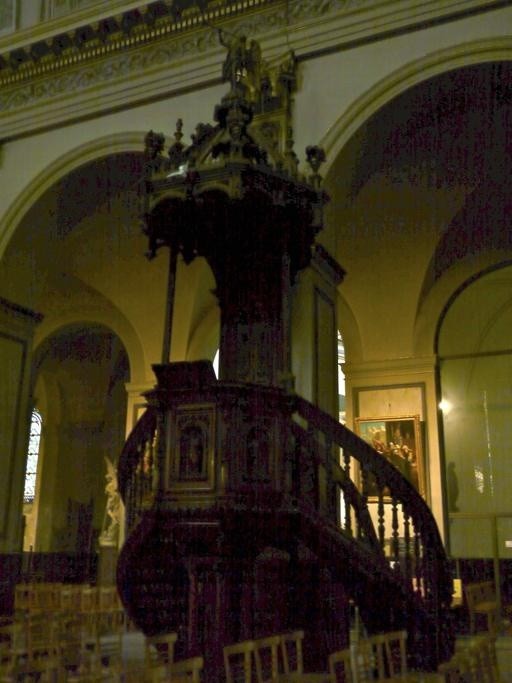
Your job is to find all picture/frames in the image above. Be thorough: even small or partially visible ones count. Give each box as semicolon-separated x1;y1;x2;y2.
353;415;426;503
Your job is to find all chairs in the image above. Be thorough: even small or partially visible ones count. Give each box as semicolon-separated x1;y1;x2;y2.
444;636;501;683
145;636;203;683
330;632;409;683
0;585;125;682
225;632;329;683
466;583;499;636
